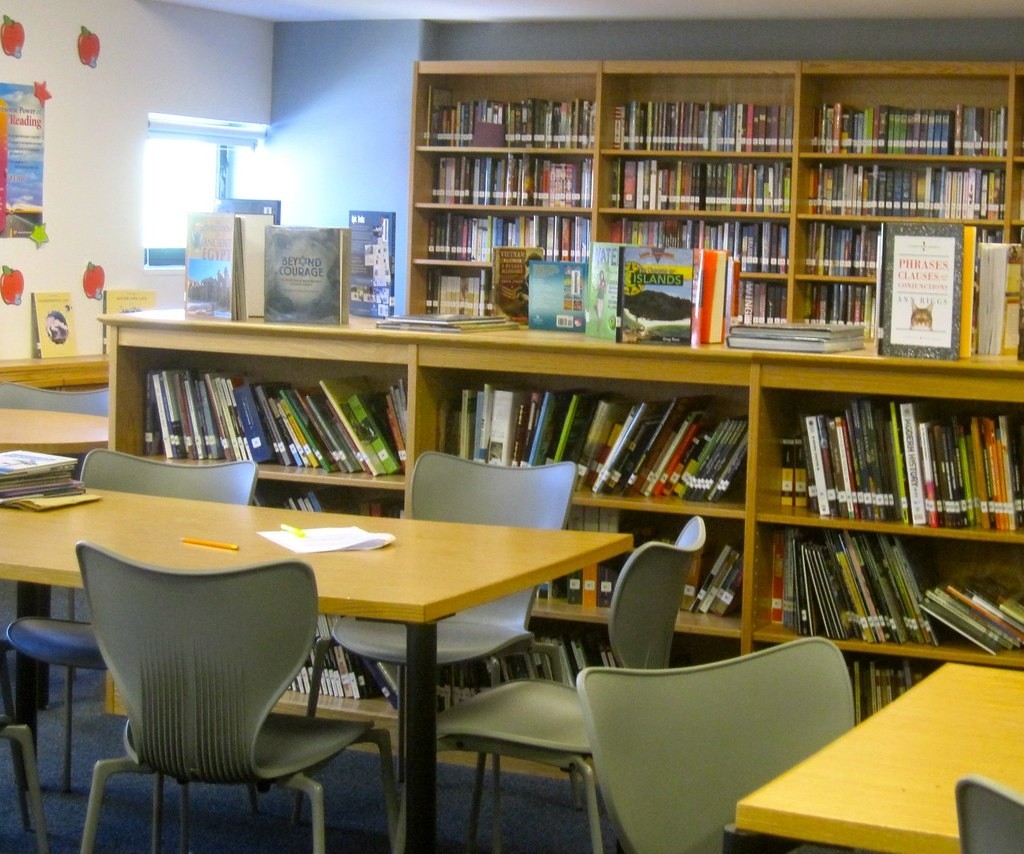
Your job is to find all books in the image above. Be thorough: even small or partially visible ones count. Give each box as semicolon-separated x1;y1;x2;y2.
770;398;1024;657
437;383;749;504
103;290;156;355
31;292;76;359
0;451;86;504
287;616;400;710
185;196;396;328
143;369;407;518
854;660;923;723
536;505;743;619
438;634;617;718
425;82;1024;362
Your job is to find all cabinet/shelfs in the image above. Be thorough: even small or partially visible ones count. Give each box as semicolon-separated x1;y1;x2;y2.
99;309;1024;784
407;52;1024;362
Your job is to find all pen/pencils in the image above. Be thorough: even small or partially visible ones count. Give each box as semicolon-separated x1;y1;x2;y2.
180;537;238;550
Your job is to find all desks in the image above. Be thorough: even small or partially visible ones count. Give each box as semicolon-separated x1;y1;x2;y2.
722;661;1024;854
0;486;633;854
0;408;109;713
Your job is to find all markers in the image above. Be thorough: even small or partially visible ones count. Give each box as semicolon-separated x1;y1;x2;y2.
281;524;306;537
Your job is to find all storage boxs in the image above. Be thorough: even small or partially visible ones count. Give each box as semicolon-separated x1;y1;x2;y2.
264;224;350;324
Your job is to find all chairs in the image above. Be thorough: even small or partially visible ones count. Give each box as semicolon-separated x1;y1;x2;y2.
74;541;398;854
289;451;580;854
577;636;855;854
437;515;706;854
5;448;259;793
0;712;49;854
954;776;1024;854
